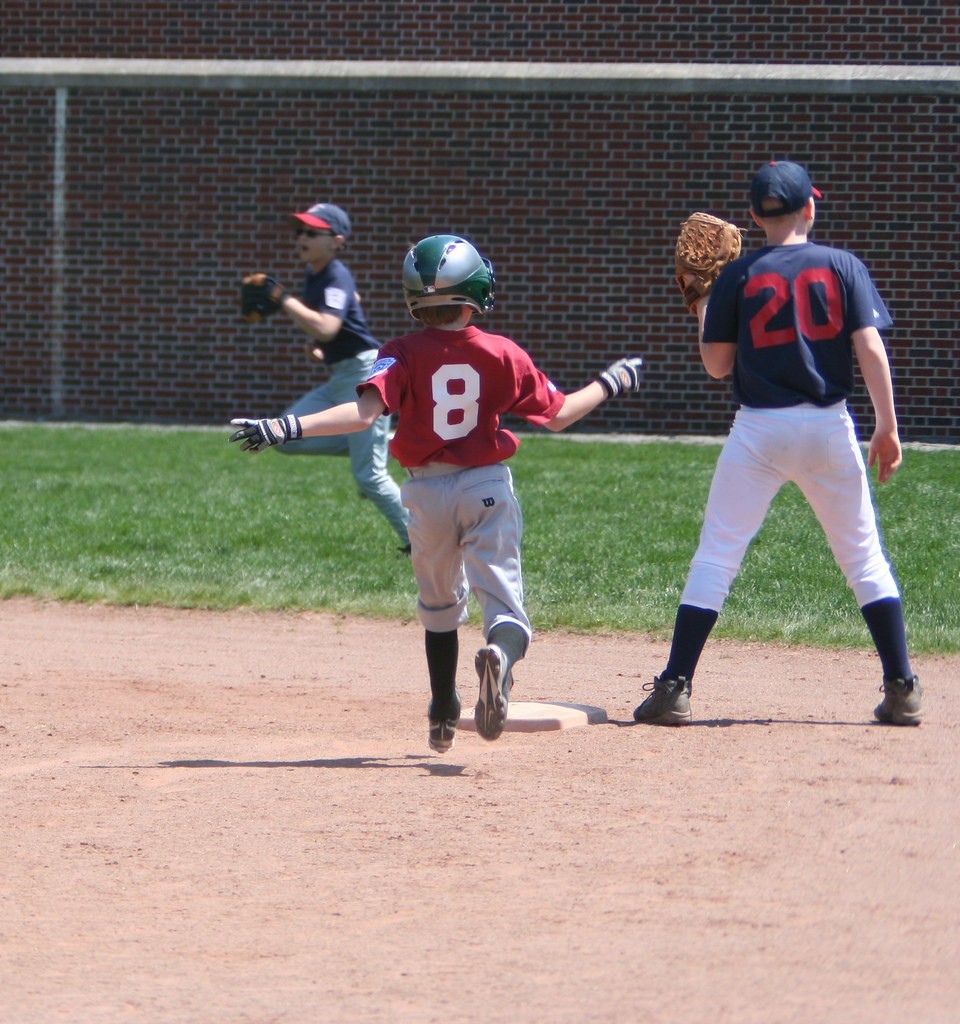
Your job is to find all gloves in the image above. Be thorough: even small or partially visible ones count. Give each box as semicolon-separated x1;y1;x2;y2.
598;357;643;400
228;412;302;457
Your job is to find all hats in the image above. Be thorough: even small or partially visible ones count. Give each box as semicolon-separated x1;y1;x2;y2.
291;201;350;241
750;160;823;218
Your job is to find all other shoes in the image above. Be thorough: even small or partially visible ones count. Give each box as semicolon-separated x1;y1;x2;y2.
428;691;460;753
475;643;514;742
395;544;413;557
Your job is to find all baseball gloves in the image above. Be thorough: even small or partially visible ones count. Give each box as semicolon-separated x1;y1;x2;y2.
674;211;743;312
241;273;289;320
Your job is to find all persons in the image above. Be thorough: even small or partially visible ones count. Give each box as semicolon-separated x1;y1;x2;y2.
634;162;922;727
228;202;644;752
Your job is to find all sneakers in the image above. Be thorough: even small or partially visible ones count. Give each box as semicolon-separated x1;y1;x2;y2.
873;675;924;727
632;672;692;727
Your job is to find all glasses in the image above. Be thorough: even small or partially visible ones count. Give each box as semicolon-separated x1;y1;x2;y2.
293;227;334;241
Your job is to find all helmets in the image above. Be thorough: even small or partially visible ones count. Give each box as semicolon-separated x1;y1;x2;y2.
401;235;495;321
